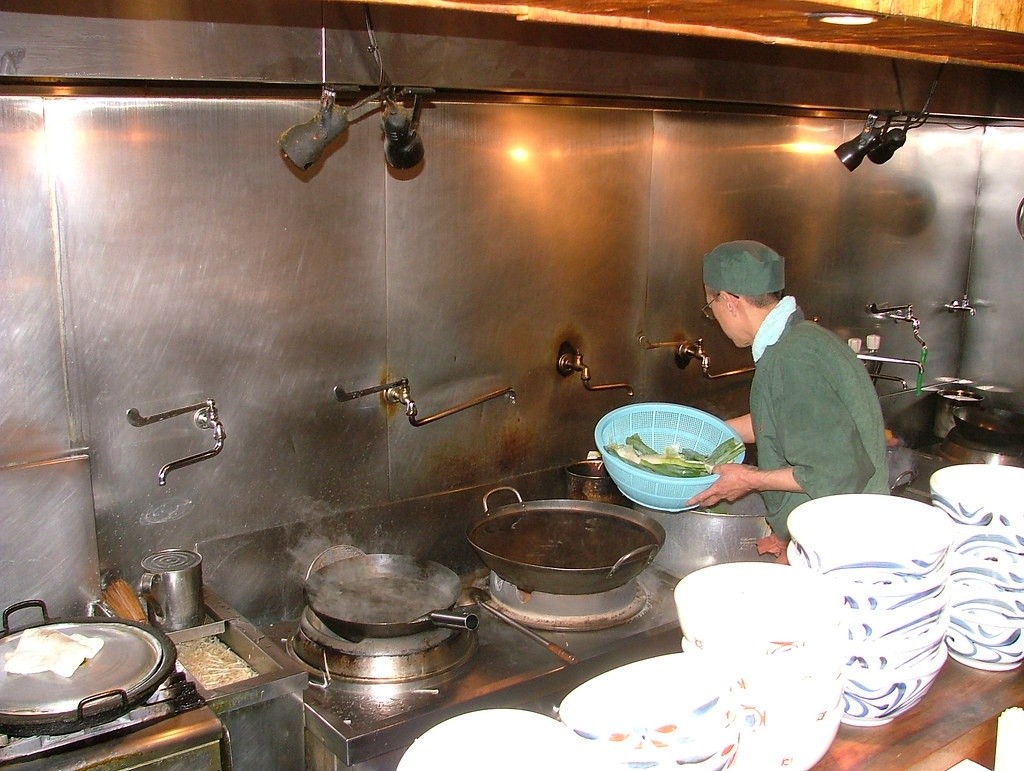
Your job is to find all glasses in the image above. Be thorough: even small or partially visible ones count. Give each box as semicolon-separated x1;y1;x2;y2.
702;290;740;320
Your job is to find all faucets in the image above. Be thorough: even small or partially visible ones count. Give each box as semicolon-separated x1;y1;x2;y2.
948;295;977;316
890;304;928;349
155;399;228;485
569;346;635;397
333;375;518;428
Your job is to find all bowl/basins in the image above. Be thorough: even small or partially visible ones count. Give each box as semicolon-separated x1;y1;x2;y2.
396;463;1024;771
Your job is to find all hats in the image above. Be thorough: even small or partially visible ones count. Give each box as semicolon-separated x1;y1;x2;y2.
703;239;785;294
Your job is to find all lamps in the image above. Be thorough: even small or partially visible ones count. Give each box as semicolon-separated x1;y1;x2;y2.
280;83;426;172
833;111;928;171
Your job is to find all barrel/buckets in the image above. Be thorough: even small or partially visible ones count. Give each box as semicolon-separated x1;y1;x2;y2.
933;389;985;440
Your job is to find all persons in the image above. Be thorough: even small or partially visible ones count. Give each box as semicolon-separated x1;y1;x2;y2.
685;241;890;564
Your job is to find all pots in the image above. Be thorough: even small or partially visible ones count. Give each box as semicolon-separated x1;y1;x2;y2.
465;486;668;595
302;553;479;644
951;407;1024;447
0;600;177;738
886;451;919;497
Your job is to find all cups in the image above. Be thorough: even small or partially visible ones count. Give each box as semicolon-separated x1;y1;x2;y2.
141;548;203;633
564;460;621;506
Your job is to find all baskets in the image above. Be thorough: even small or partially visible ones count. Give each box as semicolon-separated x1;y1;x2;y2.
595;402;745;512
